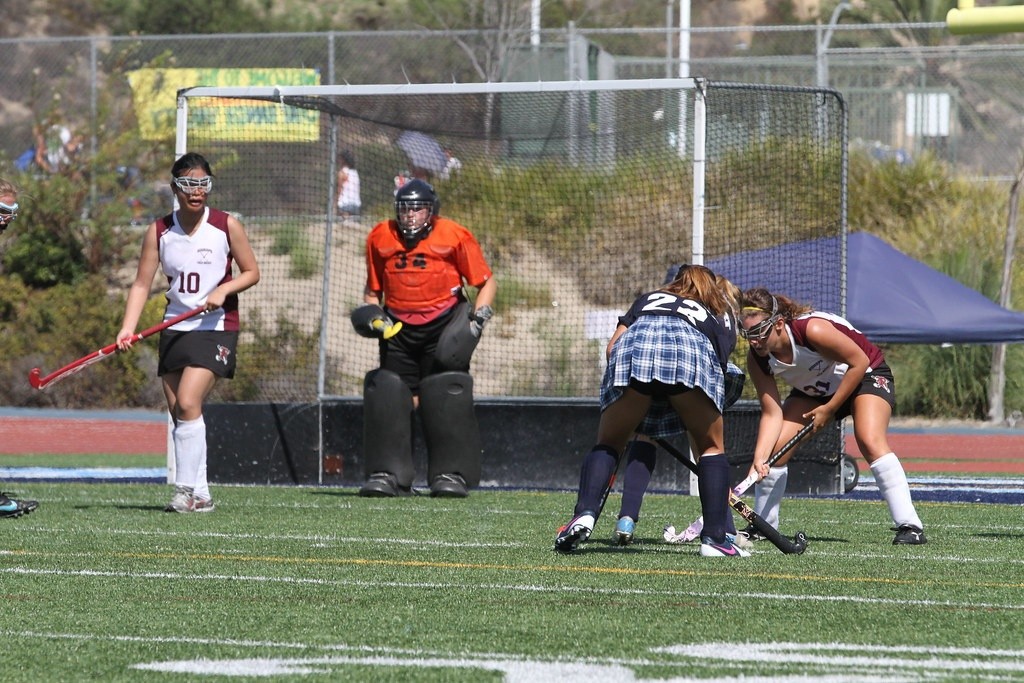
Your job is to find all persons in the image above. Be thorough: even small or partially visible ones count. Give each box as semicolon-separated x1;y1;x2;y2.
1;108;89;515
351;179;497;498
737;285;927;546
115;152;260;516
333;146;462;223
551;262;752;559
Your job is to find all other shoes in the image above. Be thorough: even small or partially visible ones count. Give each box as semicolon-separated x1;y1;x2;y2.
430;474;468;498
360;473;411;498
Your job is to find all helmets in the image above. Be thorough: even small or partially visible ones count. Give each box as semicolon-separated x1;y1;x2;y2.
394;178;441;247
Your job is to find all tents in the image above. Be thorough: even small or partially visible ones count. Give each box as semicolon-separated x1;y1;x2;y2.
696;228;1024;342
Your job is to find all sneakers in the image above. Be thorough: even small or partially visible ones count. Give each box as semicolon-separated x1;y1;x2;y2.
699;532;751;557
164;488;215;513
555;510;594;555
738;523;769;540
0;490;39;518
889;523;928;546
611;519;634;545
725;530;755;547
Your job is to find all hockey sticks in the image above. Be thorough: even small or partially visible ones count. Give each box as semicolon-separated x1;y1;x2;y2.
664;419;814;543
654;435;807;555
373;320;402;339
28;304;207;390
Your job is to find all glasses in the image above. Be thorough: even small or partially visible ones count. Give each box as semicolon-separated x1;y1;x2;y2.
174;176;212;194
739;316;774;340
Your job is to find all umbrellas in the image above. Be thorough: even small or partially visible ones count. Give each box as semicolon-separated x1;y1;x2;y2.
399;131;452;172
